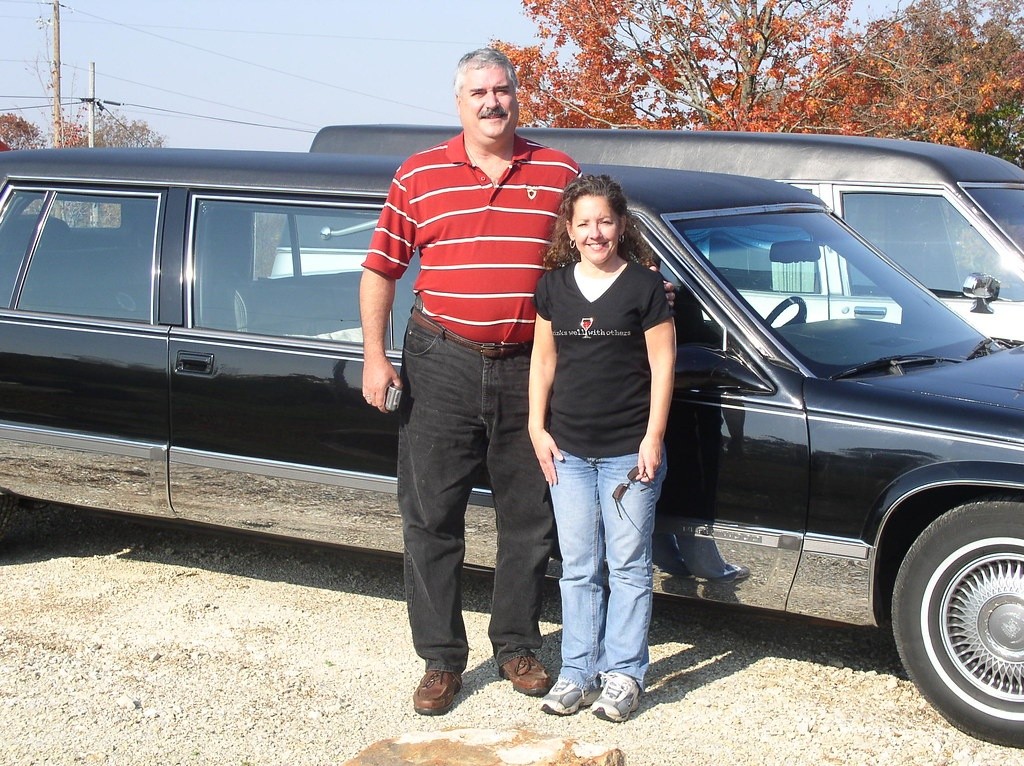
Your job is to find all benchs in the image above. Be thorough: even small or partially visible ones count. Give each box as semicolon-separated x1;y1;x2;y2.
0;244;363;341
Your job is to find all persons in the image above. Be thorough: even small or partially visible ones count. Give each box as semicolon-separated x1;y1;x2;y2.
528;173;676;722
361;47;674;717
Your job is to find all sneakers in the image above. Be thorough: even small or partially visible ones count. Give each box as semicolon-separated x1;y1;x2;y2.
670;564;697;579
719;563;751;584
413;670;463;715
592;671;640;723
541;678;603;716
498;655;551;697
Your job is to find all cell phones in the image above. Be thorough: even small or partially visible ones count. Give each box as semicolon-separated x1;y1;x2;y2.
384;383;402;411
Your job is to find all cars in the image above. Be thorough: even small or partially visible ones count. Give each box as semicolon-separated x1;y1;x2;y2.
2;146;1024;747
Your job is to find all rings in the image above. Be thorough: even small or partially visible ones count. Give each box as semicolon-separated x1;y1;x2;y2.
366;395;370;400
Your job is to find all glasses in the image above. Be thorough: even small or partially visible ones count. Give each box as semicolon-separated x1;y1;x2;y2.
612;466;655;520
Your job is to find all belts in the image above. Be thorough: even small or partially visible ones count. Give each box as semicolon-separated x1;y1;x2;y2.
411;307;534;360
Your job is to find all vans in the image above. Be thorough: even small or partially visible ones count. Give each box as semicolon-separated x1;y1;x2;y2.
269;123;1024;370
